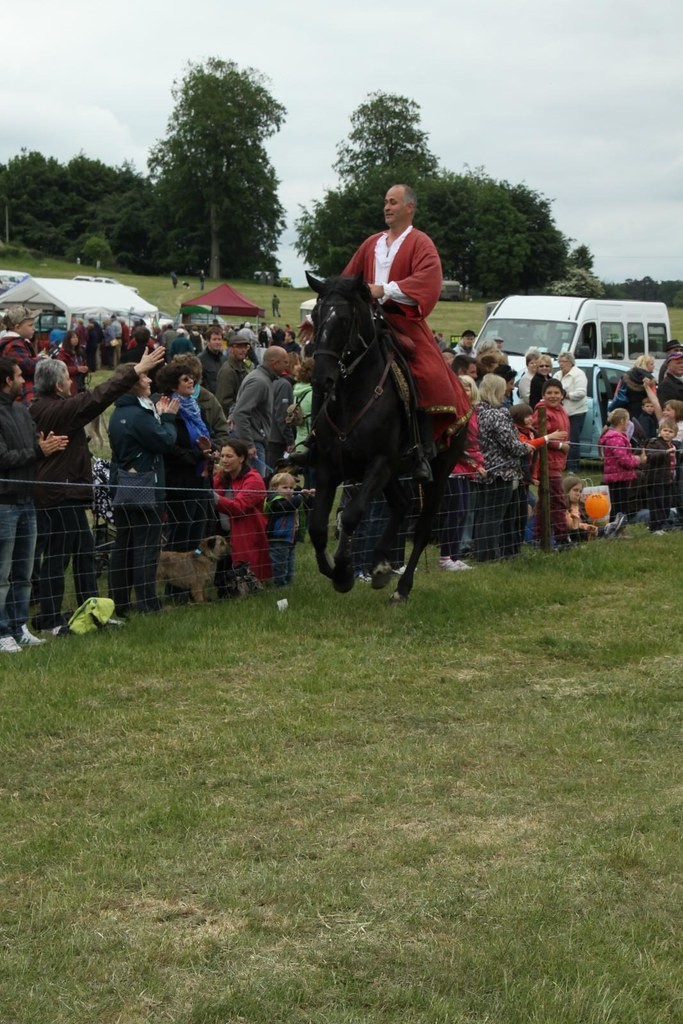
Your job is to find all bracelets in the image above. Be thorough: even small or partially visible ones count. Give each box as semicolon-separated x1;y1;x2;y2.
559;442;563;450
544;435;550;444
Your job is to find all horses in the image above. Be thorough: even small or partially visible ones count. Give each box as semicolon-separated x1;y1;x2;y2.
304;267;467;604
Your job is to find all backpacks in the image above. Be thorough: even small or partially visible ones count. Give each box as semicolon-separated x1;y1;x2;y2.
285;389;313;426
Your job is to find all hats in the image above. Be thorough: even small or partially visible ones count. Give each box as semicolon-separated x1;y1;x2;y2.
229;335;249;344
666;352;683;364
494;364;517;383
2;305;41;329
663;339;683;352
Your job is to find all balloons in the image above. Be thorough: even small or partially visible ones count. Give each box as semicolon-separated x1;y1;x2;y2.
585;493;610;519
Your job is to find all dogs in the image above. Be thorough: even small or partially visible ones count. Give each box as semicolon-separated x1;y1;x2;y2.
182;281;190;289
153;535;234;609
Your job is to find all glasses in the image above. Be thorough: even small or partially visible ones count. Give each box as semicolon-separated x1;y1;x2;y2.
539;364;552;367
179;375;191;383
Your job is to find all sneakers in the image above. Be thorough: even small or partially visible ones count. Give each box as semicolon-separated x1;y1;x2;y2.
0;635;22;652
18;624;46;646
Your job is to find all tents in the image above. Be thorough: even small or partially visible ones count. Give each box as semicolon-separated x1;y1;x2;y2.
0;276;158;343
181;280;265;334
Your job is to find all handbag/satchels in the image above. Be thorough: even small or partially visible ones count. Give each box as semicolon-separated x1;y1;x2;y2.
109;470;159;510
110;338;119;347
580;478;611;533
233;561;264;596
55;597;115;635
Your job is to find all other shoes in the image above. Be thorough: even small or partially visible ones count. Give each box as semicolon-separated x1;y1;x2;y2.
392;564;417;574
441;558;470;571
52;625;62;635
354;570;372;582
652;530;666;535
108;619;124;625
603;512;627;538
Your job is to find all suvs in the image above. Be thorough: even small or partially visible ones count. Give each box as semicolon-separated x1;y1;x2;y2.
69;275;139;295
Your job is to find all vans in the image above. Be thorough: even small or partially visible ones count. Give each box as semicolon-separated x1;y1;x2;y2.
468;295;674;411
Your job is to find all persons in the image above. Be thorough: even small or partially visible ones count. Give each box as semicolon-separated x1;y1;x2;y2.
297;183;472;478
0;257;683;654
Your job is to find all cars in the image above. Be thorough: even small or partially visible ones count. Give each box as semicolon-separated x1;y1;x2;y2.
518;361;664;463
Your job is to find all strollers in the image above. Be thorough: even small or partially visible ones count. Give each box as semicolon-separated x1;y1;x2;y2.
87;435;171;578
